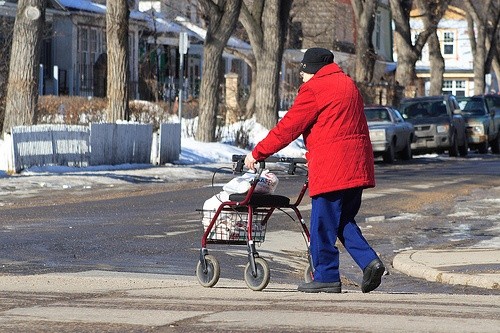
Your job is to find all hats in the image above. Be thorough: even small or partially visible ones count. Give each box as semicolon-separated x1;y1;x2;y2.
300;47;334;75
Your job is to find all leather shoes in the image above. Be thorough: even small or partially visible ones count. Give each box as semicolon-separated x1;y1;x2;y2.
297;280;341;294
360;257;385;293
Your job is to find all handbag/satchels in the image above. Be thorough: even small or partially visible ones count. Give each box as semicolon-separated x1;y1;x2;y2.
222;165;278;195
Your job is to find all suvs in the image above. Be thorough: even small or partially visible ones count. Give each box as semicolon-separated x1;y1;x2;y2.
439;92;500;154
396;94;469;158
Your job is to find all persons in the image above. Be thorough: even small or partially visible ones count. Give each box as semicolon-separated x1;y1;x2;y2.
243;46;386;294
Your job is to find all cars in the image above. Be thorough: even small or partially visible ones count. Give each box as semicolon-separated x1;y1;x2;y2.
362;105;417;163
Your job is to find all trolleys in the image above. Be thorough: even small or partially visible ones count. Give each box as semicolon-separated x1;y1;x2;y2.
193;153;316;292
